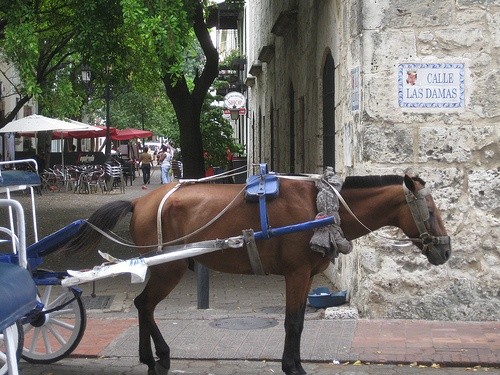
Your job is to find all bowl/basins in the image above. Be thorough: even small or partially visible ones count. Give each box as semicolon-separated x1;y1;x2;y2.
308;286;347;307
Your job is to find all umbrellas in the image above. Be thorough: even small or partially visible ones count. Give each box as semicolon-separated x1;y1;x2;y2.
22;120;125;140
0;113;88;153
108;127;152;140
16;115;103;172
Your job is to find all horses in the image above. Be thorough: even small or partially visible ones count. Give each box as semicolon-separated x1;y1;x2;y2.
62;175;452;375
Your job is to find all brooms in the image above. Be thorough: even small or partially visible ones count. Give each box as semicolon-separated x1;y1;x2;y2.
141;163;158;189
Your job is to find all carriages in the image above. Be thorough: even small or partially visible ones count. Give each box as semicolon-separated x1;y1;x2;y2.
0;158;451;375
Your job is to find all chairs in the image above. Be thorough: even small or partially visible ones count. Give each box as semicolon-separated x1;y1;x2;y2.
170;161;183;178
42;160;133;194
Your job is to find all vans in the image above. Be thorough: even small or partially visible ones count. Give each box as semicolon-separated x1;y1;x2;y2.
139;142;176;161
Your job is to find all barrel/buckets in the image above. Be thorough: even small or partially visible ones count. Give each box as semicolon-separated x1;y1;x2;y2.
232;160;247;184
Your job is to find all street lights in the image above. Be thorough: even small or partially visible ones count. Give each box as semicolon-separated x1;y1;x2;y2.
130;89;159;148
80;57;133;161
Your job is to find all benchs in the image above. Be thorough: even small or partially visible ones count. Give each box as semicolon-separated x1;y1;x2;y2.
0;198;38;333
0;159;41;191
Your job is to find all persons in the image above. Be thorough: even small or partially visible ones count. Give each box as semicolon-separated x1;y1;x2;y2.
139;147;152;189
159;145;172;186
152;145;163;165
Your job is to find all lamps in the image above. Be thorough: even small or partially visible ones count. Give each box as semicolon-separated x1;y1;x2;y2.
229;106;251;120
